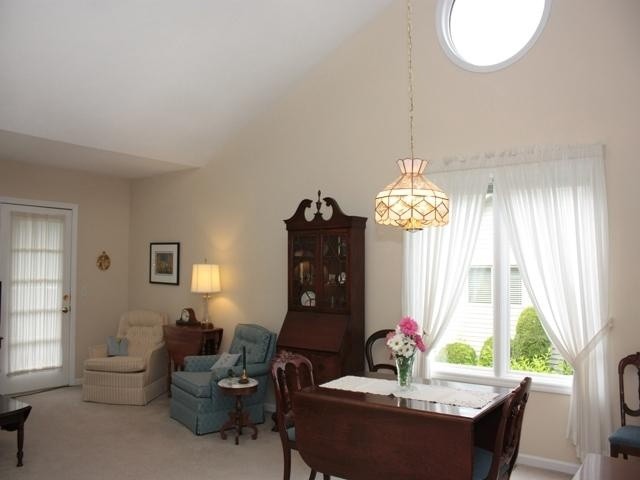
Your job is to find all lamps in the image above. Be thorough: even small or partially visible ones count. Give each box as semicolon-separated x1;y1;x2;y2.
374;92;450;231
191;263;221;328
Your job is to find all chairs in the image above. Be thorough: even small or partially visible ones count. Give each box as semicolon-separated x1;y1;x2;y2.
272;352;329;480
608;351;640;460
472;377;532;480
367;329;395;374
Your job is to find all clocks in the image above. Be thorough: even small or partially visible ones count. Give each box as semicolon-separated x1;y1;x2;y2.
176;309;200;327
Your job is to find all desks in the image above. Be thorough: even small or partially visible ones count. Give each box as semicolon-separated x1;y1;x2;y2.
217;377;259;445
162;323;223;397
0;396;31;470
296;370;513;480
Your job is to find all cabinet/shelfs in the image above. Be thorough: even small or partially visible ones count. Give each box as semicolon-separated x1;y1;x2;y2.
276;190;368;384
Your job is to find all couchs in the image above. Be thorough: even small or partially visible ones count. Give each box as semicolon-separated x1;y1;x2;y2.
83;314;169;406
170;323;278;435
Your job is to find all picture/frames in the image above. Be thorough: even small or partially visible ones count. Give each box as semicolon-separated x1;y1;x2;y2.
149;242;179;285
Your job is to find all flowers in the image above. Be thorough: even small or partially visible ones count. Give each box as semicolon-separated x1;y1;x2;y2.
385;316;432;385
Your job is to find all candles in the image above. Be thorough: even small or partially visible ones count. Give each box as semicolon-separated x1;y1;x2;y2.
242;346;247;370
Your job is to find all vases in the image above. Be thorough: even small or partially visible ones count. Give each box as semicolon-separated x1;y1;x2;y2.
395;357;415;392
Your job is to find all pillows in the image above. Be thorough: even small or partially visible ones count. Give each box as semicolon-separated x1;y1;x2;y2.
106;336;128;356
211;352;241;370
